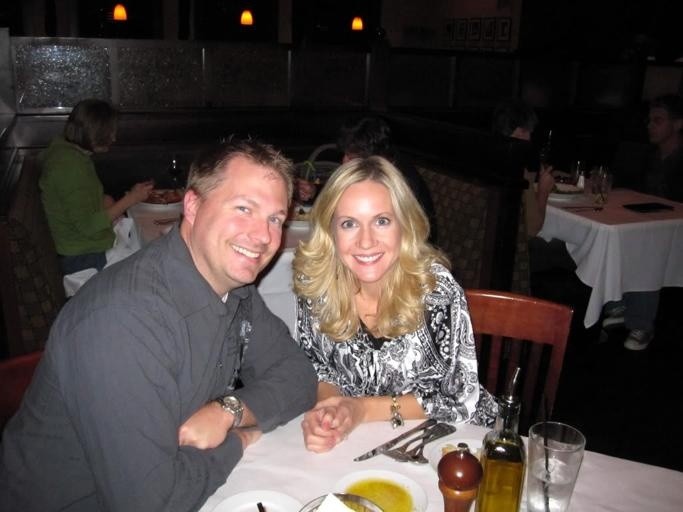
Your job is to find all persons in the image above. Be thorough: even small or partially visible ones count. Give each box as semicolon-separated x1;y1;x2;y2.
600;95;682;353
2;136;321;512
289;116;440;248
493;96;609;346
32;99;156;275
290;153;506;446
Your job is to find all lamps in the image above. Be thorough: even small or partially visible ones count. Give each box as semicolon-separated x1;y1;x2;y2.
349;14;367;31
111;1;127;21
239;7;254;27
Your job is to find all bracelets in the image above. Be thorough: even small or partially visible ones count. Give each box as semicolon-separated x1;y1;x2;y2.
388;392;406;430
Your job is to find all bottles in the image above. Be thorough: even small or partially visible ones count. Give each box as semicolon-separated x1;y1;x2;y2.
473;366;526;511
436;440;482;512
573;160;585;192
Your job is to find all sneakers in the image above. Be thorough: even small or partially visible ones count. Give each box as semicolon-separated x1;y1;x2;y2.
623;324;656;351
601;303;628;331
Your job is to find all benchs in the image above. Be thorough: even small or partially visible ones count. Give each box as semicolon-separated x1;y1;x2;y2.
0;145;71;358
386;150;533;305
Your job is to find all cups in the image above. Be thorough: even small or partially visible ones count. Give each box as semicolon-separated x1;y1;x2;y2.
590;170;610;205
526;420;586;512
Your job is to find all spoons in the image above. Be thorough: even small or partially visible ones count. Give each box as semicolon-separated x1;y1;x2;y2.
410;427;429;468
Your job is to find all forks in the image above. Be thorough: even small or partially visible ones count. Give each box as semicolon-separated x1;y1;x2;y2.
381;421;453;462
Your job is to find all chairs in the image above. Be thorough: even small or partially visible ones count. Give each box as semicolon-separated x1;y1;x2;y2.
0;347;46;434
451;283;574;445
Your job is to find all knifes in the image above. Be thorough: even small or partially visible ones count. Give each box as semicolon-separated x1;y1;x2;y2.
354;418;438;462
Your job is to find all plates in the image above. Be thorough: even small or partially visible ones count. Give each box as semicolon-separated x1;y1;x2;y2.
329;469;429;512
284;207;315;232
136;197;182;210
533;180;585;201
212;488;306;512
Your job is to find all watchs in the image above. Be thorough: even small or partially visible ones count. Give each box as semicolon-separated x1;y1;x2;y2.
214;391;243;430
